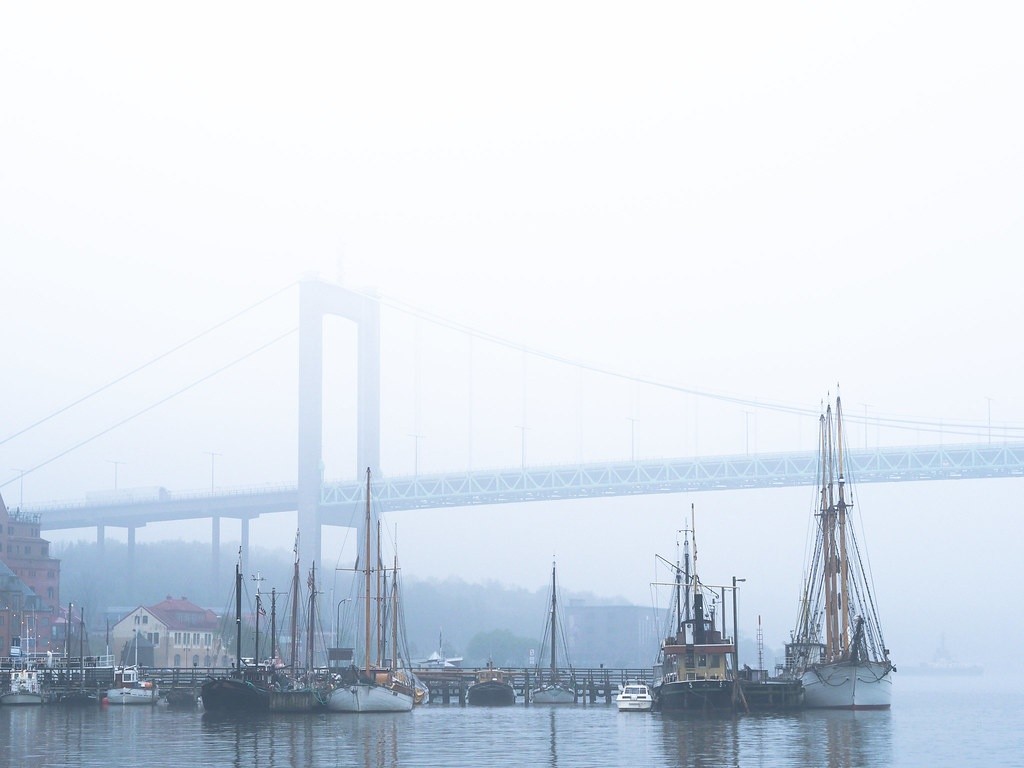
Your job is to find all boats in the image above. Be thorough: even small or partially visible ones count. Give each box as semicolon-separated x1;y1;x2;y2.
647;502;760;717
615;683;654;711
465;650;518;707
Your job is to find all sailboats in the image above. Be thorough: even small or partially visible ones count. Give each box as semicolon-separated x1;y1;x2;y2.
779;381;898;710
0;464;427;714
528;549;578;703
